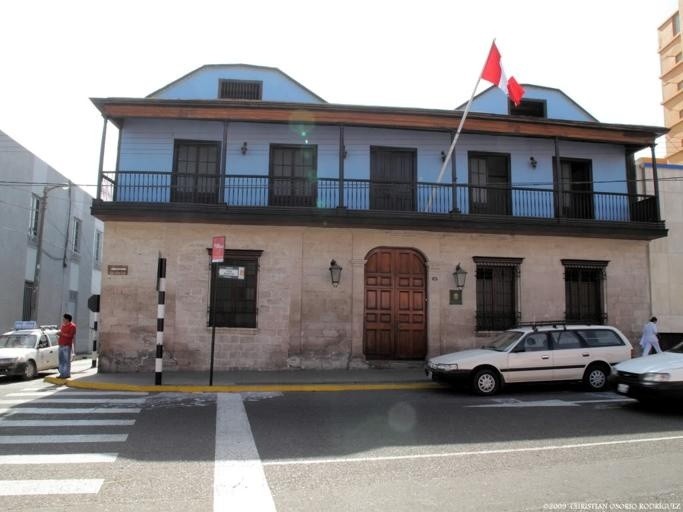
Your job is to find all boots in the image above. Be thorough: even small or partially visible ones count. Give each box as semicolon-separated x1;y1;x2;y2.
56;375;70;378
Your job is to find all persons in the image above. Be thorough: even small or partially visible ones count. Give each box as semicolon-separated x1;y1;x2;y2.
56;313;76;378
639;317;663;356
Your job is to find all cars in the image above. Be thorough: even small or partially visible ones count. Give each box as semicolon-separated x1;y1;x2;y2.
0;321;75;380
425;320;683;402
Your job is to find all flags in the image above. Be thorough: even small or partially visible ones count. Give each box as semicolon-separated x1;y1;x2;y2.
480;42;525;108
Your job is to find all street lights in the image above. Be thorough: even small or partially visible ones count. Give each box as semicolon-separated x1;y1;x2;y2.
31;182;70;320
